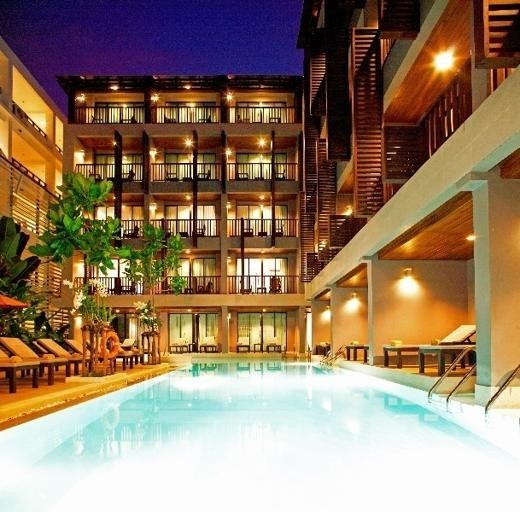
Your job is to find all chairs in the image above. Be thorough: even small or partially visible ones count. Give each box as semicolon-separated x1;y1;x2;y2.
363;345;369;364
87;115;286;294
237;337;281;352
419;344;476;376
383;325;476;368
169;336;216;352
345;345;363;360
0;336;144;393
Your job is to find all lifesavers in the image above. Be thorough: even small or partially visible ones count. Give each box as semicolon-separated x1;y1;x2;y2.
100;332;120;360
